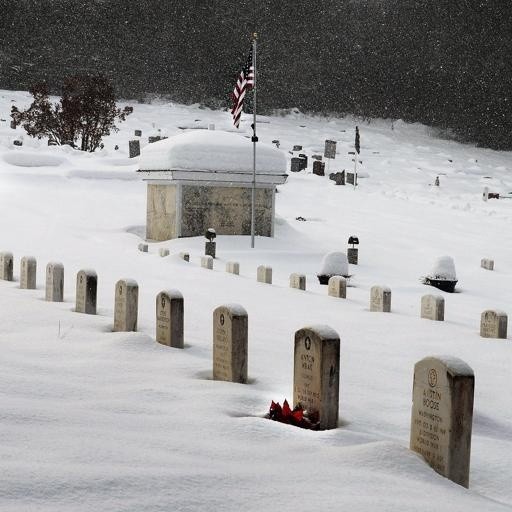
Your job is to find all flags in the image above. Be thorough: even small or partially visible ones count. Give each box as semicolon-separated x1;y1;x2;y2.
354;125;360;154
231;42;256;129
